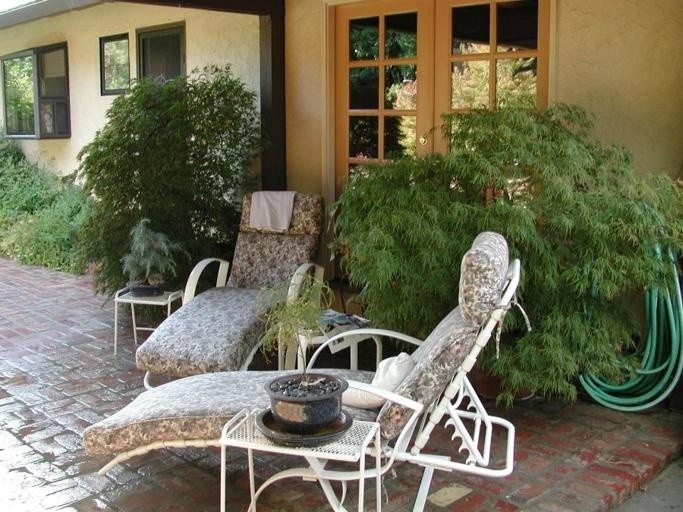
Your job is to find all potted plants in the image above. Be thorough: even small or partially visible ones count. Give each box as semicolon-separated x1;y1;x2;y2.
255;272;353;446
119;216;192;296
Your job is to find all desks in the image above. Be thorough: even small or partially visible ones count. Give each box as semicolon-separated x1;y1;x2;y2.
221;408;382;511
113;285;184;355
278;308;384;372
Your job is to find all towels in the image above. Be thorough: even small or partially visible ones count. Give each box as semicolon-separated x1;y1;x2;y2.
250;190;298;233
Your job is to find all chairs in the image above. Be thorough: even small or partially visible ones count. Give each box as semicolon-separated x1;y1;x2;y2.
135;188;324;391
82;230;521;512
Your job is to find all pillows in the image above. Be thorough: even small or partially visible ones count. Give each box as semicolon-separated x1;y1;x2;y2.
343;351;413;409
